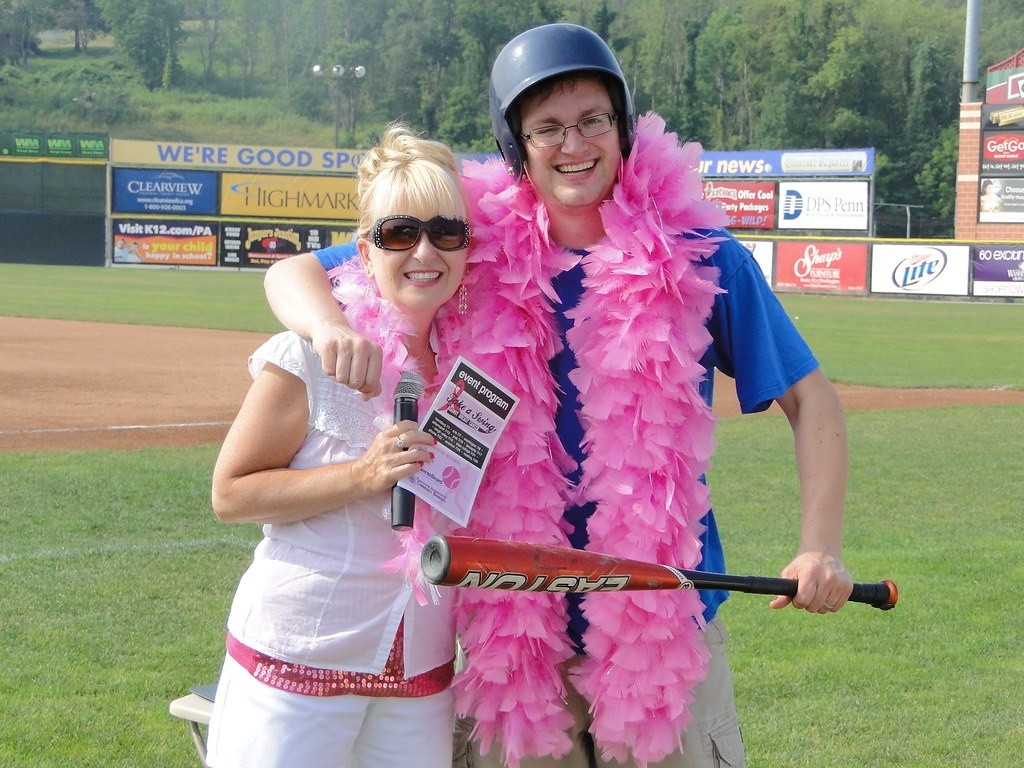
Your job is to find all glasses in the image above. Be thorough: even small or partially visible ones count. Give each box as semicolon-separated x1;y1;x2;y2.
520;113;623;149
361;214;471;253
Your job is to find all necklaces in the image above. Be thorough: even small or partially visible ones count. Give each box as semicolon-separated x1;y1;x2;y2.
418;340;430;369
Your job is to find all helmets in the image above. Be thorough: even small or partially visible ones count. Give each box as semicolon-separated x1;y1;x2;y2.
487;24;634;186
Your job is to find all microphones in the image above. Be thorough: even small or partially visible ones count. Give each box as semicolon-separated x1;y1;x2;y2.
391;371;424;531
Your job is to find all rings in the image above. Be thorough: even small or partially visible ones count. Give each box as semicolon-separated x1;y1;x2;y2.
396;435;405;450
822;602;834;611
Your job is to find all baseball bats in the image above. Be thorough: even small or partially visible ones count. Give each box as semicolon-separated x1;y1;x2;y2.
419;531;901;615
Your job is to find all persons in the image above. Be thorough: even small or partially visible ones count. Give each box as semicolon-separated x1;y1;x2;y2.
265;26;850;768
206;125;471;768
